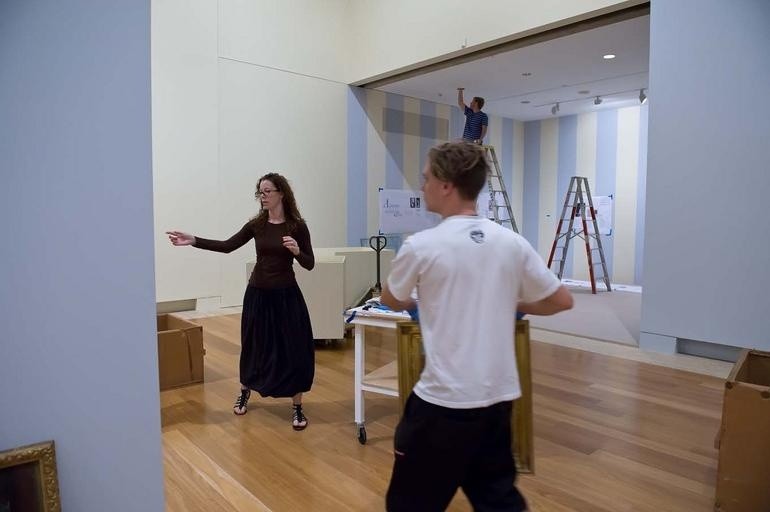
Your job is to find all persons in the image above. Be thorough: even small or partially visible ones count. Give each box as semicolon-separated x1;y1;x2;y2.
164;172;315;431
456;87;488;145
379;141;574;512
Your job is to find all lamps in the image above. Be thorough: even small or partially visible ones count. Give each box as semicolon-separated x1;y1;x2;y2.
534;87;648;115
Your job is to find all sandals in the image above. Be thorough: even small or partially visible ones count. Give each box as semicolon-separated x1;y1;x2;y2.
291;403;307;431
233;388;251;415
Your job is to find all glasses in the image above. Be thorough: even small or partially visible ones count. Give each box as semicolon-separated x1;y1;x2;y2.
257;189;279;195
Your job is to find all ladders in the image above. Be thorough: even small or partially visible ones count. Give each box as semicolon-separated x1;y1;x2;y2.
475;144;518;233
547;176;611;294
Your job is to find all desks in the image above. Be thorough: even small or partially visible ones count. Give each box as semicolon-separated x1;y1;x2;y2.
246;246;412;446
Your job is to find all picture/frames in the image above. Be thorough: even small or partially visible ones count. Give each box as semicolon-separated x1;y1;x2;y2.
396;320;535;475
0;440;61;512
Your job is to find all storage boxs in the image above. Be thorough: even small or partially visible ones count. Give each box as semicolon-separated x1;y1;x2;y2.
157;313;205;391
713;349;770;512
